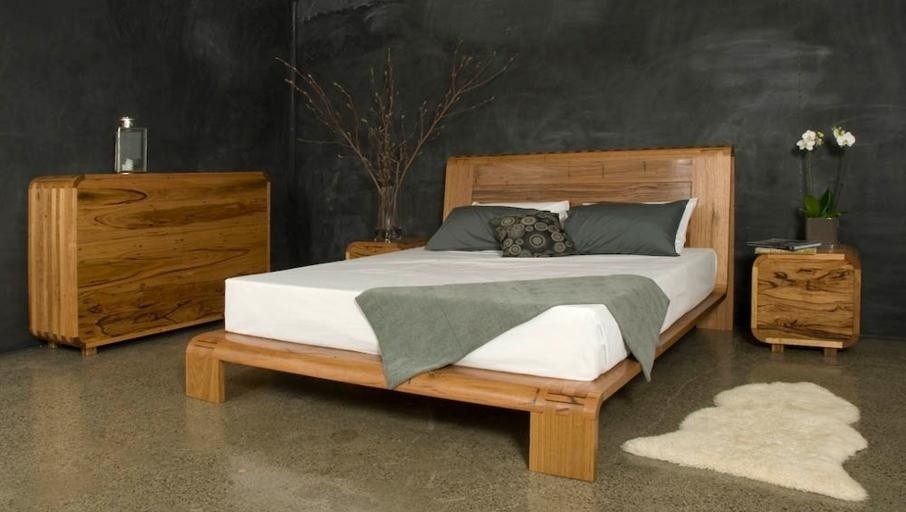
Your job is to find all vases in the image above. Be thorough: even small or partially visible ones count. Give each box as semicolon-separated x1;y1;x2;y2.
375;186;403;245
804;217;841;248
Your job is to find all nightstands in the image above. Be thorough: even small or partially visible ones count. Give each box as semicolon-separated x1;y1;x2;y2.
750;244;861;358
344;238;429;263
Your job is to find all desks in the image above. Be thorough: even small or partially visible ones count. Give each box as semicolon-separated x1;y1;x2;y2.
28;171;270;356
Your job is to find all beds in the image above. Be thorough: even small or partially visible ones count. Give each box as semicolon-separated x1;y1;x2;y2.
185;147;735;482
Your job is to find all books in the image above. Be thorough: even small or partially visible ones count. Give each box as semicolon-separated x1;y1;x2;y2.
754;246;819;255
746;237;824;251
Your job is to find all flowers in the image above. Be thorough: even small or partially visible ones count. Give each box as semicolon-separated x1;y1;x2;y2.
796;124;856;216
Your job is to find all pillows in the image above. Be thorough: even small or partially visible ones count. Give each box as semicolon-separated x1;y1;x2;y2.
425;207;538;250
471;199;569;232
582;197;699;256
563;198;688;257
488;213;578;257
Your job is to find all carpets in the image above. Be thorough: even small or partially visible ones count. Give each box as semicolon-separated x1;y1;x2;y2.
619;379;871;501
